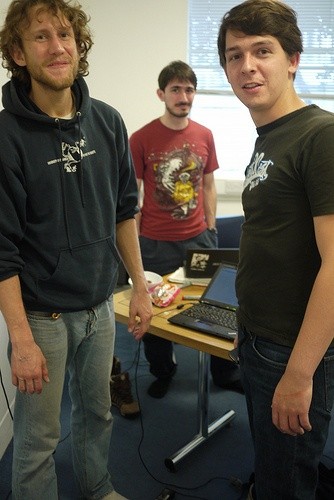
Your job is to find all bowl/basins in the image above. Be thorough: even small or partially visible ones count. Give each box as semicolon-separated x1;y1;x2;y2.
127;271;163;291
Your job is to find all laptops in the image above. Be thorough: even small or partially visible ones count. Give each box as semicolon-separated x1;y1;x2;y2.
183;248;241;278
168;264;238;342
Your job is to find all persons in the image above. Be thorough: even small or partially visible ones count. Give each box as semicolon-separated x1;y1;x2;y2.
109;356;139;420
216;0;334;500
0;0;153;500
129;60;242;397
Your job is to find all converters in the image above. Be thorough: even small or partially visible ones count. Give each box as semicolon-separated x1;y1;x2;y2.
153;488;174;500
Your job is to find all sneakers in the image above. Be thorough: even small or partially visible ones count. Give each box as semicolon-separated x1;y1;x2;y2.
111;375;142;420
146;363;177;400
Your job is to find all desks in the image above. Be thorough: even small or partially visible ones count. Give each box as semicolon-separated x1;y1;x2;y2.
113;273;237;468
